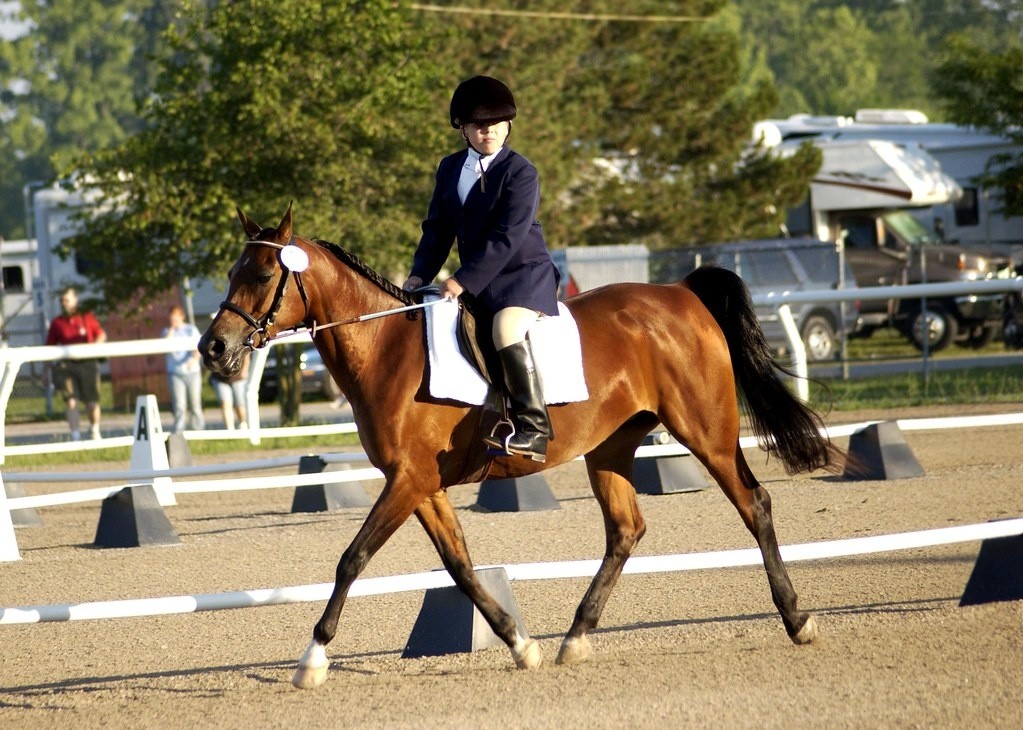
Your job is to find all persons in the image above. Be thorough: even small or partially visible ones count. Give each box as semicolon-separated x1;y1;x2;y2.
402;75;561;464
161;307;205;431
208;353;251;430
45;289;107;442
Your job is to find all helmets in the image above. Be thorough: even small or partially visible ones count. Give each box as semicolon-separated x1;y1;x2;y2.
450;74;517;128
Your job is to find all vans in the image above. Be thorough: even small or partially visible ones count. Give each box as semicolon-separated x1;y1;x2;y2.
735;105;1022;351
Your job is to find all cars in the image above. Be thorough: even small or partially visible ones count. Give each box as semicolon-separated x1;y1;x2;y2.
258;327;342;403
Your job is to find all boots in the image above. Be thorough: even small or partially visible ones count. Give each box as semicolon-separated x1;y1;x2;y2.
482;339;550;464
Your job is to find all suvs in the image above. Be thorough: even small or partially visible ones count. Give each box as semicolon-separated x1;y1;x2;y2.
646;235;861;364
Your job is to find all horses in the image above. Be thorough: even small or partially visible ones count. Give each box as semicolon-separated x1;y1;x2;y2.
197;197;873;691
